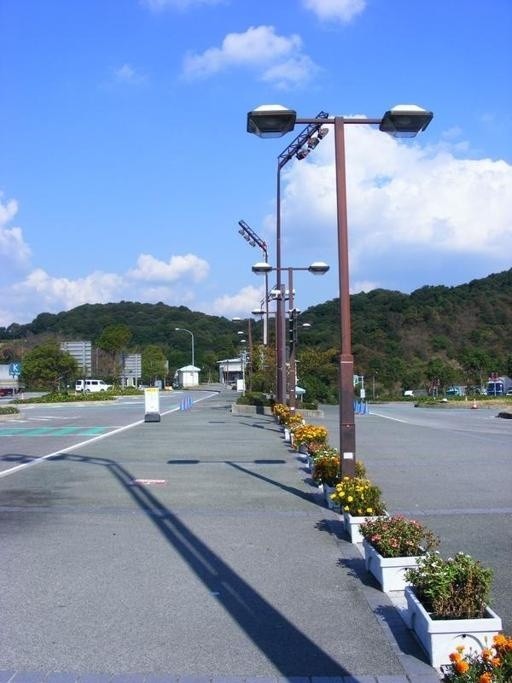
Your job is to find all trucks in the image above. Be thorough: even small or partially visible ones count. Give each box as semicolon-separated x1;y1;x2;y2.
0;365;25;396
487;375;509;396
446;386;468;396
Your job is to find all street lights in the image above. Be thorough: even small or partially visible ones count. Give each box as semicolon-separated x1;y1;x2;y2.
236;219;271;347
175;328;194;366
250;309;301;416
232;104;433;482
251;261;330;405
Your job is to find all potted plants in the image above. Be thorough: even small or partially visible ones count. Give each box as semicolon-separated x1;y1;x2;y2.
273;403;390;545
404;551;500;668
358;512;440;594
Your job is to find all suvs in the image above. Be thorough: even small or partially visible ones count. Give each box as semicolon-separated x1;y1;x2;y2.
75;380;112;392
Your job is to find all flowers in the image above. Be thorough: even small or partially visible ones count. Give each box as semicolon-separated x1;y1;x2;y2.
440;633;512;683
